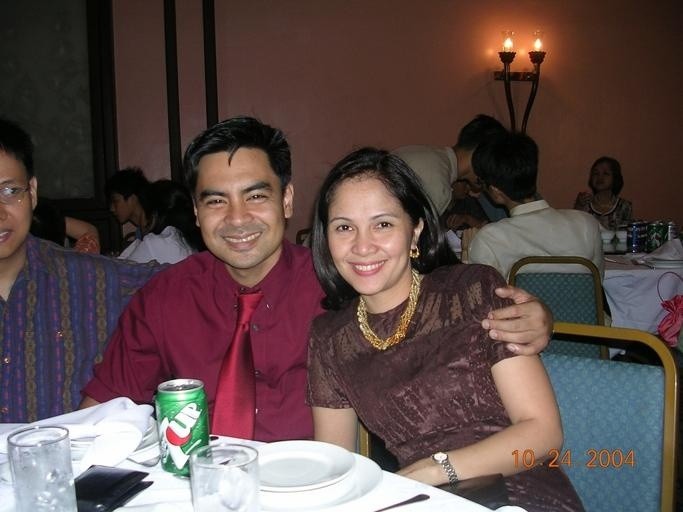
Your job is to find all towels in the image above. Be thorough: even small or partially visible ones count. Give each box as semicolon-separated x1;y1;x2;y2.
643;237;683;261
0;396;154;478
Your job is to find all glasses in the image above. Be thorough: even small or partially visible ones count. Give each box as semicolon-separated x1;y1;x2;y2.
1;182;32;205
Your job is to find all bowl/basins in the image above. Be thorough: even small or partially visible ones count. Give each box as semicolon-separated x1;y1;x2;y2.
602;244;628;253
601;230;627;243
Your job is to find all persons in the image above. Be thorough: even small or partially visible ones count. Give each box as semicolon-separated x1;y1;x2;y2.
575;156;633;230
306;147;584;512
28;166;198;265
0;121;172;424
389;114;604;283
79;116;552;441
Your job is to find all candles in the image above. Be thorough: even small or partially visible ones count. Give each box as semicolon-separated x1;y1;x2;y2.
492;37;548;57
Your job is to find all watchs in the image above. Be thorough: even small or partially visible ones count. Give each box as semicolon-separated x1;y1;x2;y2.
432;452;458;486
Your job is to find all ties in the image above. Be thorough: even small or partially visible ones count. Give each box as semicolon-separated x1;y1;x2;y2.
209;290;272;442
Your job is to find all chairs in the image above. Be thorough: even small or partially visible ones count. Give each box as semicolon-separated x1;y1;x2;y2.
355;412;369;459
508;256;612;360
540;321;679;512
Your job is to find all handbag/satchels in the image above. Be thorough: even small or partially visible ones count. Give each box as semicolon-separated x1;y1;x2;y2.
436;474;510;511
654;270;683;348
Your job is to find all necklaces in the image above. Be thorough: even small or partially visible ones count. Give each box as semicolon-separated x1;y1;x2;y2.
356;269;421;352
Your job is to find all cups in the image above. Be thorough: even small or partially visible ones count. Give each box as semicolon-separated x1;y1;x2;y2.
6;426;79;511
187;444;256;510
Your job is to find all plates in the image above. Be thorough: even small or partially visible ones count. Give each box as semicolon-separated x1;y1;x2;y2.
255;440;386;512
133;413;161;454
648;259;683;269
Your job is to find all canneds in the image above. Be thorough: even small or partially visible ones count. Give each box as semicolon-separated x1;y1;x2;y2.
628;220;681;255
155;378;210;477
